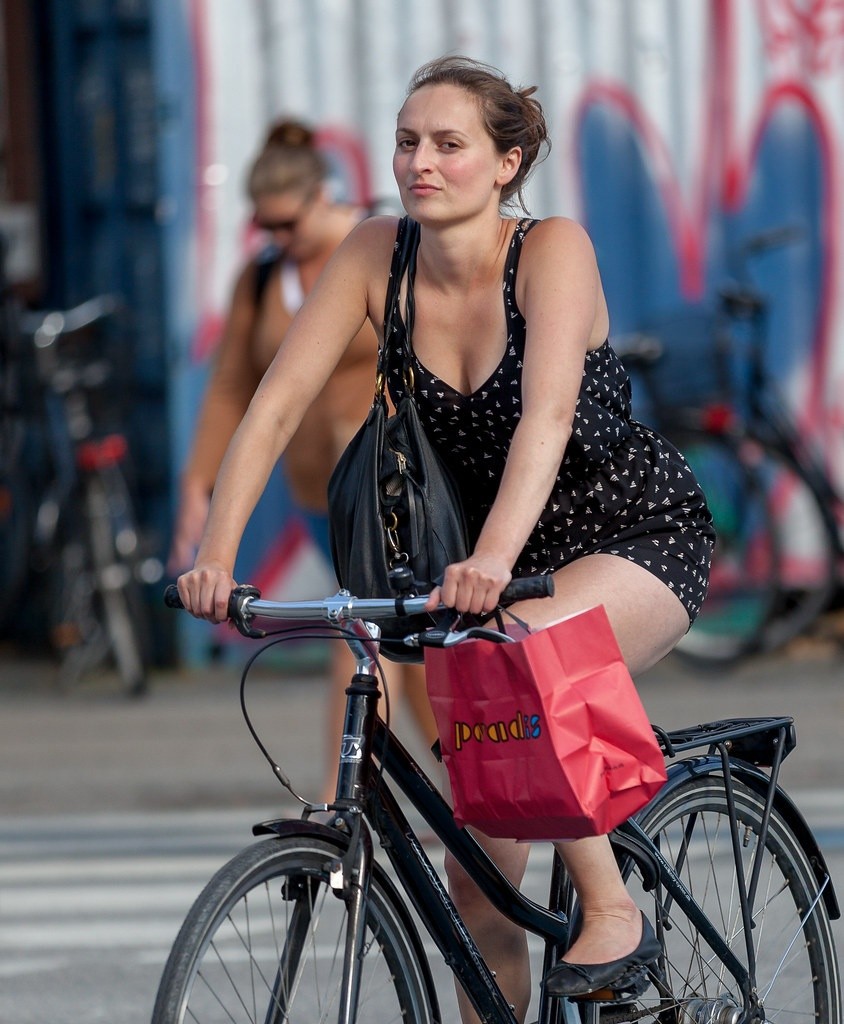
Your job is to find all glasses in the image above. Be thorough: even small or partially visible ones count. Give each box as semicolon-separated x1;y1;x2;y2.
252;189;318;233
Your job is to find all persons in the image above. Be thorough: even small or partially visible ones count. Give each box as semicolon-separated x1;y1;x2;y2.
169;119;444;823
169;55;716;1024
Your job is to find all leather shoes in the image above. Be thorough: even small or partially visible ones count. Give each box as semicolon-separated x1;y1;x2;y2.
539;910;662;997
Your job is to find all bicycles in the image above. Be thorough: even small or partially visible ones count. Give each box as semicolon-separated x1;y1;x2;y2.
605;220;844;673
139;568;844;1023
1;288;151;696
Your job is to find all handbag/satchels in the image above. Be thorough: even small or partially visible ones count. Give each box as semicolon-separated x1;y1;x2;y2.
423;596;667;839
325;215;470;664
